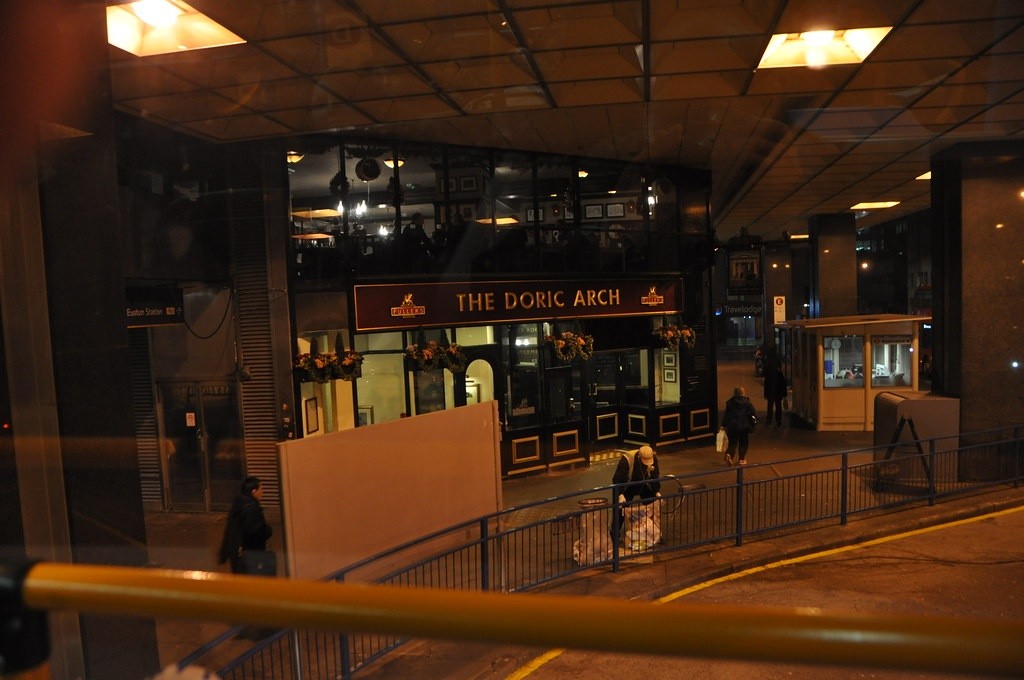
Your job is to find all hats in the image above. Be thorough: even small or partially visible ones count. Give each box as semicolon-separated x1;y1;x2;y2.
639;446;654;465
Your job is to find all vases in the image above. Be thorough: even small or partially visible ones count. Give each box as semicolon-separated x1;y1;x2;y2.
428;358;447;369
297;369;315;383
406;358;421;371
337;364;362;380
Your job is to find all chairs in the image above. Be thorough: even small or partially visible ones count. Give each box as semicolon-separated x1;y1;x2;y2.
387;239;426;274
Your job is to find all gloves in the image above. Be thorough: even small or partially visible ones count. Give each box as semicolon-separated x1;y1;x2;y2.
656;492;662;502
617;494;626;509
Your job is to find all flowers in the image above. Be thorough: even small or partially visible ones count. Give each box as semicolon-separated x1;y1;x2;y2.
402;339;468;374
295;350;365;385
553;331;594;364
651;324;697;353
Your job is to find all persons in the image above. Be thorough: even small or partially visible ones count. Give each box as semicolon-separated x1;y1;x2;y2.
399;214;439;274
719;385;756;466
552;219;576;248
610;446;661;545
217;476;277;641
755;352;788;425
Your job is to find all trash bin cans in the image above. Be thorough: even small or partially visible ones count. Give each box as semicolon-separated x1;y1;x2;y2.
577;498;611;564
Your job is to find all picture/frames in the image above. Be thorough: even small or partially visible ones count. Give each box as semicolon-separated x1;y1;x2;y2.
459;204;476;222
663;353;677;367
663;368;677;383
604;202;626;219
358;405;374;427
440;205;456;224
526;207;545;223
304;397;319;435
460;175;478;192
584;204;604;220
562;205;583;221
439;176;458;195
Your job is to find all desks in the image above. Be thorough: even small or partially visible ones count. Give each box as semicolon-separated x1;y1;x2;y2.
599;247;626;272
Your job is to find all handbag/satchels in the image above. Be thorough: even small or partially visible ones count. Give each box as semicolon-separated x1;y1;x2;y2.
716;429;728;452
748;409;759;425
242;549;276;577
783;397;788;409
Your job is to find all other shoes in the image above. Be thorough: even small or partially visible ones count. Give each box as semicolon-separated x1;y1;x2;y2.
725;454;733;467
739;459;747;465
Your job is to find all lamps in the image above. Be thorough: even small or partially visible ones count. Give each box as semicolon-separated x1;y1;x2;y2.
287;151;304;163
384;159;406;169
